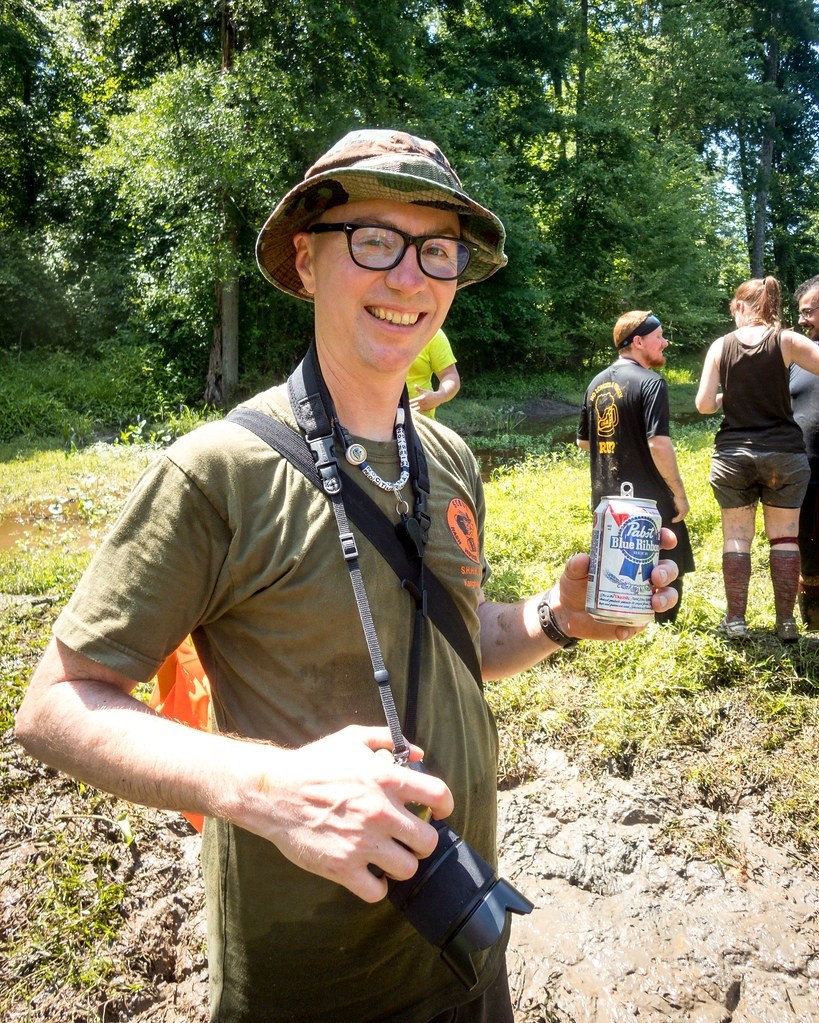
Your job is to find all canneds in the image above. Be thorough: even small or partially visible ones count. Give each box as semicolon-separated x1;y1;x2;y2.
584;482;664;627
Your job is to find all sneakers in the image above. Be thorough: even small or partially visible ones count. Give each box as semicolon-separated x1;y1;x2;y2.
775;616;799;640
718;616;745;636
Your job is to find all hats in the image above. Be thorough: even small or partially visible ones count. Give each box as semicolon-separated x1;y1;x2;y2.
255;129;509;303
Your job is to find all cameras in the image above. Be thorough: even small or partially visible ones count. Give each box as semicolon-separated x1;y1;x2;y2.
365;761;534;992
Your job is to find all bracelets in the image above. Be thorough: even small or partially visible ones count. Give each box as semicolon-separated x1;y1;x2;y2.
538;585;582;653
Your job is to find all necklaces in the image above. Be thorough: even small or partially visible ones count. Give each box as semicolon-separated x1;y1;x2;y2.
310;346;427;559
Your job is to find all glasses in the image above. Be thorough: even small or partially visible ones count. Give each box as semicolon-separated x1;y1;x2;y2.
308;223;478;280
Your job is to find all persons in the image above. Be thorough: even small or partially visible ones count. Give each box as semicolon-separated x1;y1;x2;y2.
787;271;819;632
694;275;819;645
575;310;697;629
404;327;460;425
11;130;680;1023
